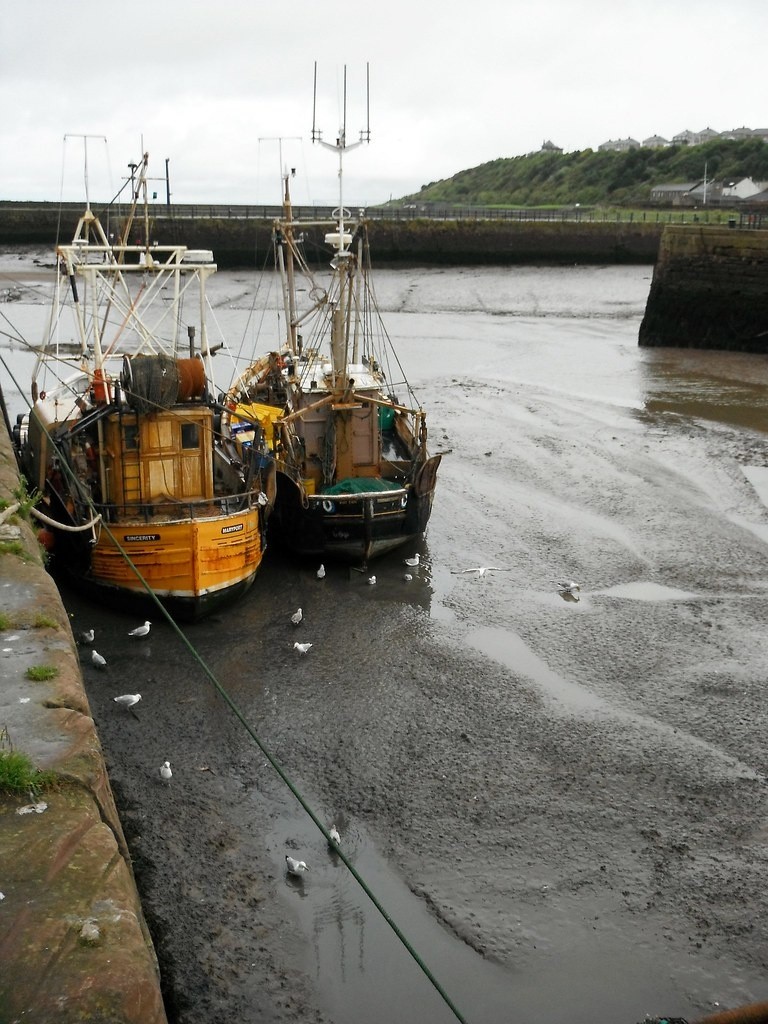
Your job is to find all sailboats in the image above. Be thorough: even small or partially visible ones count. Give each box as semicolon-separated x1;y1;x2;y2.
221;58;442;573
10;149;269;620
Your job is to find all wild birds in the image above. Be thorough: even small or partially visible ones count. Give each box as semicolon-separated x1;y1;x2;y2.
113;694;142;711
82;629;95;644
293;642;313;656
316;564;325;578
91;650;106;668
404;574;412;581
158;761;172;786
290;608;303;624
128;621;152;637
328;825;340;848
285;855;310;876
368;576;376;584
557;581;580;592
403;553;420;566
